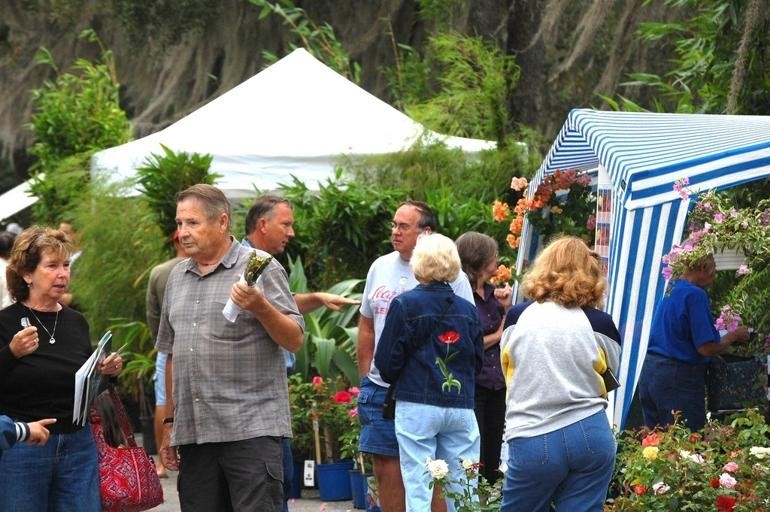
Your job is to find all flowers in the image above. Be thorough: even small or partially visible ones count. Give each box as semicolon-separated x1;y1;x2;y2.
436;331;461;394
288;374;373;475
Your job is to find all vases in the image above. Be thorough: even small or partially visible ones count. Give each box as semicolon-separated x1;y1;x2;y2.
290;462;381;511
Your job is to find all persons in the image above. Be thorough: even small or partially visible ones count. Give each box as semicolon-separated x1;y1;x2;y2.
356;199;513;512
142;183;361;512
636;252;752;443
497;236;622;512
0;218;124;512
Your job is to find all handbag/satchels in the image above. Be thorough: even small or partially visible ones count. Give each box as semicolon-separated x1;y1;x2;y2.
91;387;164;512
382;386;395;419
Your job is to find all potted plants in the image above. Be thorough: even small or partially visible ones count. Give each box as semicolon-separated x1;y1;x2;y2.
107;320;160;457
